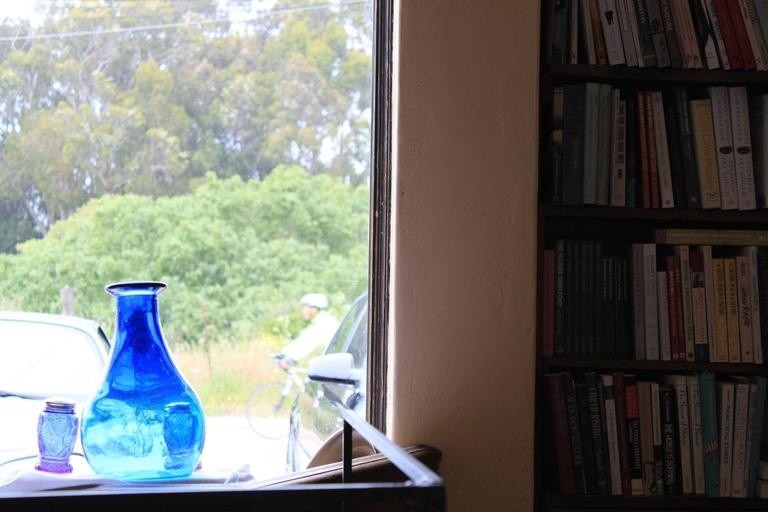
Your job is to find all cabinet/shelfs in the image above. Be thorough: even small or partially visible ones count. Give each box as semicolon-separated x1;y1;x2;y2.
535;0;768;511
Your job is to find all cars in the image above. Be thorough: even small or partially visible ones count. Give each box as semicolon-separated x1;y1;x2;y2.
2;310;110;463
287;291;367;472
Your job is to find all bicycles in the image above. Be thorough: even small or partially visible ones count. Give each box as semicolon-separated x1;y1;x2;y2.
246;354;304;440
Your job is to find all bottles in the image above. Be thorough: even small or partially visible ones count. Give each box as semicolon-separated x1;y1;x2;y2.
38;282;205;484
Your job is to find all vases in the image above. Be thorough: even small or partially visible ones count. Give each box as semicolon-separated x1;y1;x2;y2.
33;280;207;485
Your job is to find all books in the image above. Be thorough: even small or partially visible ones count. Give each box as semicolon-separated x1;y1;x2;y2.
541;231;767;364
553;83;766;210
543;372;768;497
557;1;768;71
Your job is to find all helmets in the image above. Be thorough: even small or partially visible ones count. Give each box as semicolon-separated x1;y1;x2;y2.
300;292;328;310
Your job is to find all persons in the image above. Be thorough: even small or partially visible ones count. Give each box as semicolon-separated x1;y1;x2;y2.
274;293;342;369
